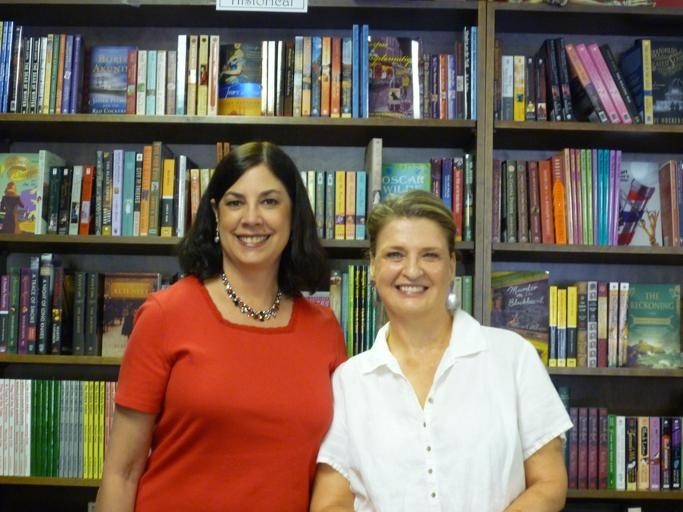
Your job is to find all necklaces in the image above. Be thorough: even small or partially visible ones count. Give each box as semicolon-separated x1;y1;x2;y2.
218;272;284;321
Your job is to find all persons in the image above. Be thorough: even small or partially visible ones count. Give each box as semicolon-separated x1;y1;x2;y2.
309;189;575;512
93;140;349;512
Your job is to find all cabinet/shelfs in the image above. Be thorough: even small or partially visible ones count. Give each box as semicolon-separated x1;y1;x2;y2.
485;2;678;497
0;0;482;490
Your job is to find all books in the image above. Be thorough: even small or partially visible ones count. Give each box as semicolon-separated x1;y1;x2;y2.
551;382;683;489
0;20;479;121
492;38;682;128
463;276;472;313
0;251;187;359
489;268;683;371
0;133;219;239
300;262;389;360
492;147;683;251
0;377;119;481
299;169;368;241
380;153;477;243
452;274;462;318
